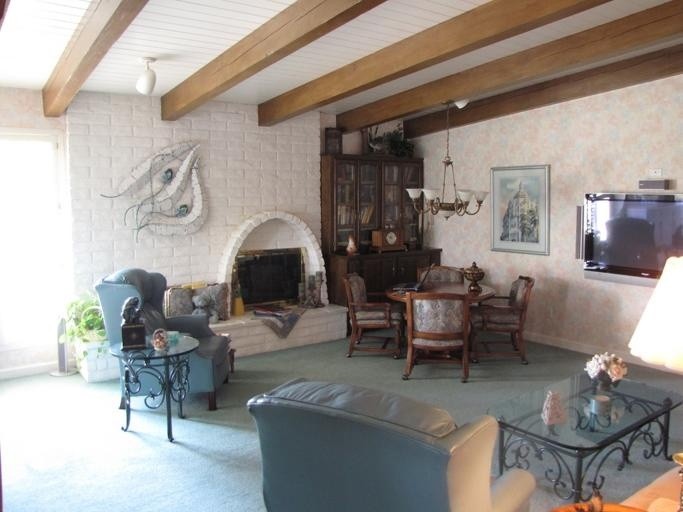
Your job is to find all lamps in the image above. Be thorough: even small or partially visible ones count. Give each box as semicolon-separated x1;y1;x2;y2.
405;99;489;222
627;255;683;512
135;56;158;97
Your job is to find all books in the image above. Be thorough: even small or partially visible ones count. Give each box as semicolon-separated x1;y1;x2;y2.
335;163;418;242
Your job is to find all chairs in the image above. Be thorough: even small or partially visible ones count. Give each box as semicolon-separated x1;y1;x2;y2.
246;377;536;512
342;265;535;382
96;268;231;411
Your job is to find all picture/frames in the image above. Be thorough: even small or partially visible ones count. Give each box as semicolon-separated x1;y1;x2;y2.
490;164;549;256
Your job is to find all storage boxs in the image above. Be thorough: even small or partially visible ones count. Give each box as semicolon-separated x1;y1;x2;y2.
121;323;147;351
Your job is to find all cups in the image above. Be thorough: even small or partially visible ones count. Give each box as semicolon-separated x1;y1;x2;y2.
590;394;612;417
166;330;178;346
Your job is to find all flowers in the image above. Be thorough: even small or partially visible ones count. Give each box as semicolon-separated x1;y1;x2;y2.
583;351;626;387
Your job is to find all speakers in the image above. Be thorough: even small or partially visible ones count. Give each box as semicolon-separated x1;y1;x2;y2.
636;178;669;192
575;204;583;261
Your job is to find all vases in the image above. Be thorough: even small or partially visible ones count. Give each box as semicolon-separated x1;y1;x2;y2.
596;379;612;392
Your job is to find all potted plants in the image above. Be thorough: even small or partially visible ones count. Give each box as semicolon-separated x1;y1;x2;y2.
82;315;100;340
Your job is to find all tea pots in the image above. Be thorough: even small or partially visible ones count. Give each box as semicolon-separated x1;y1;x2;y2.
151;328;168;352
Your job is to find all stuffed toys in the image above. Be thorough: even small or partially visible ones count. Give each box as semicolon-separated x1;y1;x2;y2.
190;294;219;324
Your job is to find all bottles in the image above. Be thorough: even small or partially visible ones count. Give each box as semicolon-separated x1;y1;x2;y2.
541;390;565;425
345;233;358;256
232;297;245;317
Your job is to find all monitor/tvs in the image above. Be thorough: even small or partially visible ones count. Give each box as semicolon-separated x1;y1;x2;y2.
583;191;683;287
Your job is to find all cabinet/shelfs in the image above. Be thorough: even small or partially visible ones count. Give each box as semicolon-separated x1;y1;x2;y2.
320;152;442;305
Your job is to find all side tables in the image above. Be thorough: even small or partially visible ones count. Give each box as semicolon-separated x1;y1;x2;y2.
109;336;200;442
